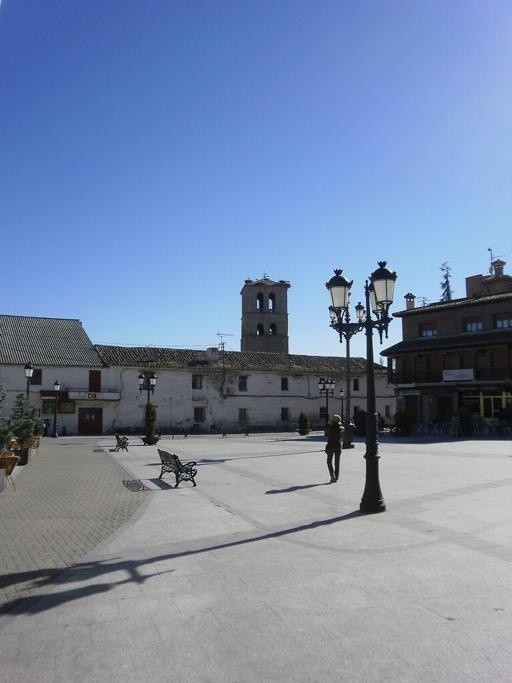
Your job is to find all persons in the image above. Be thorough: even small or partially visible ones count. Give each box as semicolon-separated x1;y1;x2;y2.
324;415;345;483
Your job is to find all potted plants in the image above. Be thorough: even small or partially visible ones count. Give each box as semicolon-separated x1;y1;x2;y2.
139;401;161;445
296;411;312;437
0;393;44;465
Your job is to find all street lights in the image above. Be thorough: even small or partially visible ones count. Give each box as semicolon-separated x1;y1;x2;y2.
317;259;398;515
24;360;35;402
52;379;62;438
137;370;160;446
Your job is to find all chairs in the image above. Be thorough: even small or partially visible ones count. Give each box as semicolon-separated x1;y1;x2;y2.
0;455;22;492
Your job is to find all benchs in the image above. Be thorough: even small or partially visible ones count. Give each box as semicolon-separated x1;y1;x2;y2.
114;432;129;451
157;449;197;488
